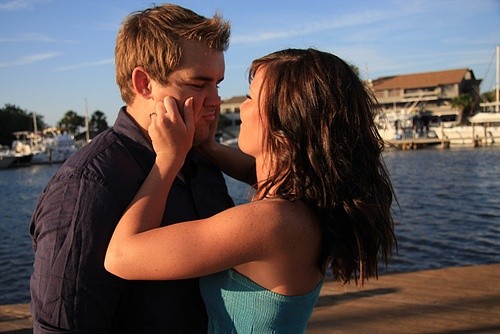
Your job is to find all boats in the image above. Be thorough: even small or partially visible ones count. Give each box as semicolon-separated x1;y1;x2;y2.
0;128;78;169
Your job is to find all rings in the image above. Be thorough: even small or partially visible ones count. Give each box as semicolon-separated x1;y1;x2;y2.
149;112;157;117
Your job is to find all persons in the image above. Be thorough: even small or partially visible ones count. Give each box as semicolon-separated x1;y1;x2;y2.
29;4;235;334
103;48;401;334
